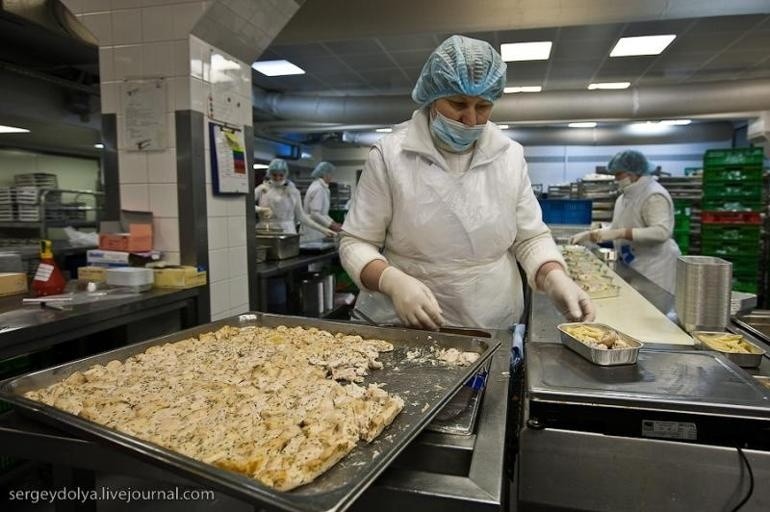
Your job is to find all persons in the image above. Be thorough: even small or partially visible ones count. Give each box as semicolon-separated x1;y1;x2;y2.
336;31;600;334
298;160;344;244
254;157;339;237
565;147;683;297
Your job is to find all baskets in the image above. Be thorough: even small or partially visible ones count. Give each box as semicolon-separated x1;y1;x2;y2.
674;147;762;292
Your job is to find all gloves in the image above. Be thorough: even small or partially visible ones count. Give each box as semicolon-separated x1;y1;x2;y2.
595;228;626;241
378;266;444;332
262;208;272;219
544;269;595;323
570;231;590;245
321;228;337;238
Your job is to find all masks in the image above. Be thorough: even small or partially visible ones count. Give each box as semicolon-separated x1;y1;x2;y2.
618;177;632;192
270;178;286;187
430;111;486;154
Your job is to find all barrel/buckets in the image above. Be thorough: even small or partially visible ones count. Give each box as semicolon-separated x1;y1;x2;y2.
302;271;334;310
294;279;325;314
